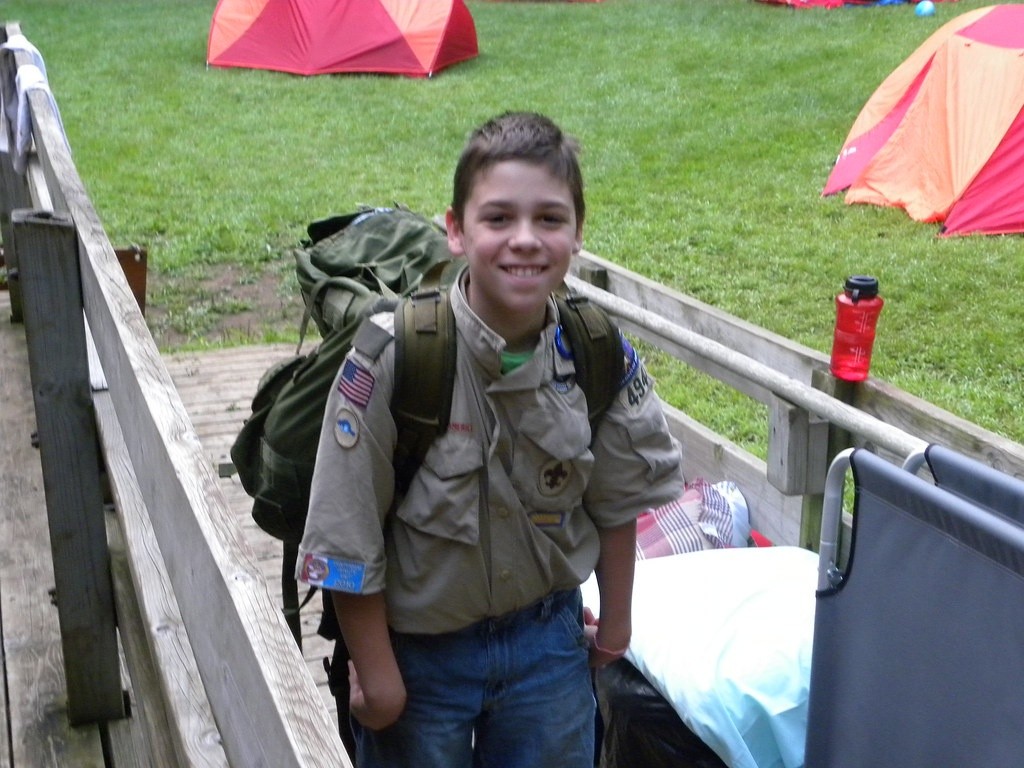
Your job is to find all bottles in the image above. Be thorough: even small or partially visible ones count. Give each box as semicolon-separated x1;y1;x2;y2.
832;275;883;381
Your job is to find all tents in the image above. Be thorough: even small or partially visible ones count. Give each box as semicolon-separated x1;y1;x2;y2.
204;0;479;82
824;0;1024;238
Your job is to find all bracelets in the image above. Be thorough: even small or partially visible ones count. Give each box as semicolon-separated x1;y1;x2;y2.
594;630;631;655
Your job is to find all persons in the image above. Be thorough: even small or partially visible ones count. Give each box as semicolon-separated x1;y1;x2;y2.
293;112;685;768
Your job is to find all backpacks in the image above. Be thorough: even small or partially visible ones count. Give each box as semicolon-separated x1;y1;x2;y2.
228;202;627;544
